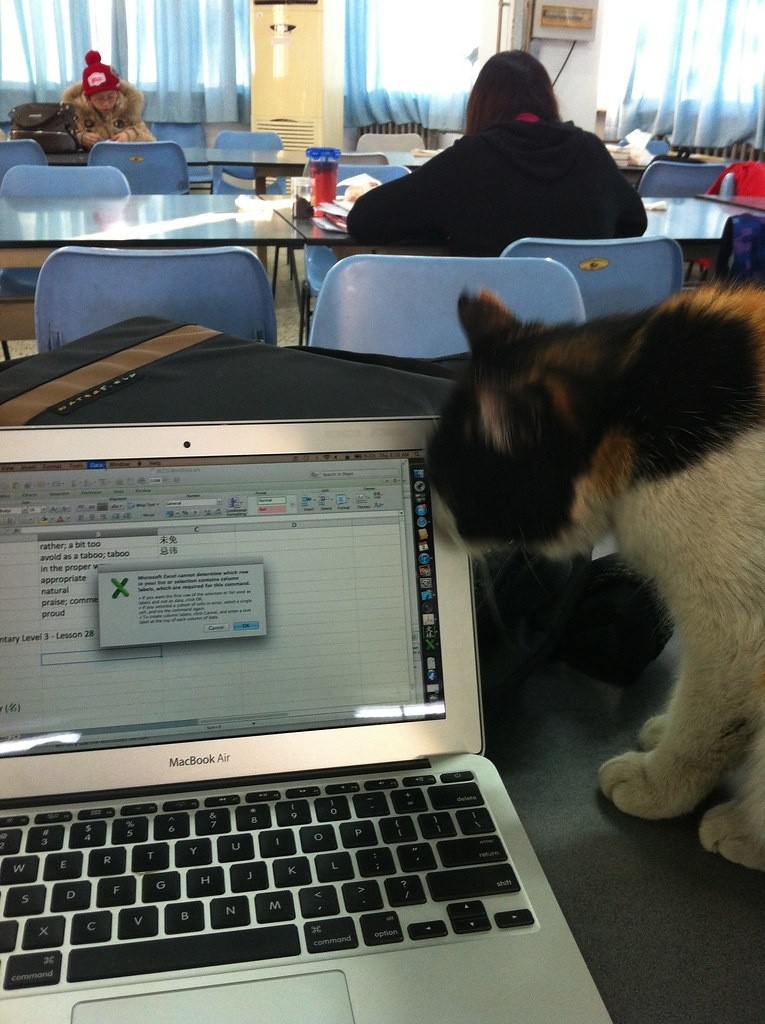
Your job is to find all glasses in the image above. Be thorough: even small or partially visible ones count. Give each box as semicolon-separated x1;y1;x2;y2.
89;92;121;104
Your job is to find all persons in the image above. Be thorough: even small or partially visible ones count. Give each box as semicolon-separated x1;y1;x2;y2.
346;50;648;258
60;50;156;148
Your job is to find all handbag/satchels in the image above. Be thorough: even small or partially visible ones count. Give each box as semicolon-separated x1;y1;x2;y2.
8;102;80;154
692;161;765;269
712;213;765;290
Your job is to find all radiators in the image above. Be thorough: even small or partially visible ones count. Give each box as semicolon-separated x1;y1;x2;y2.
685;139;765;162
358;123;439;149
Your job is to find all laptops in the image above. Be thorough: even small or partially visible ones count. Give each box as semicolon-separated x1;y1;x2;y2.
0;414;613;1024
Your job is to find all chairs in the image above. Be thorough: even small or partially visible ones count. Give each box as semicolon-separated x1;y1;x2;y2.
356;132;426;152
619;139;669;156
0;154;765;360
0;123;287;296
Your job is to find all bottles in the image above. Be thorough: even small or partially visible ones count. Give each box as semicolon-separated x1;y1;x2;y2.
305;146;341;217
289;178;316;217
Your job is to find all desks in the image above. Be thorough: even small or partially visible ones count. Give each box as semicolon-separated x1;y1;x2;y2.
199;148;747;273
482;529;764;1024
260;194;765;284
45;147;207;165
696;194;765;211
0;194;308;248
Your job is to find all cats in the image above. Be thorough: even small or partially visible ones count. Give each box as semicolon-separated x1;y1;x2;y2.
424;286;765;875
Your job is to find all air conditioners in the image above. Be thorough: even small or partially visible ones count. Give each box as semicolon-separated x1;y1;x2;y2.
251;0;325;194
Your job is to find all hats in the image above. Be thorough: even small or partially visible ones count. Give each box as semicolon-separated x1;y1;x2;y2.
82;50;121;96
